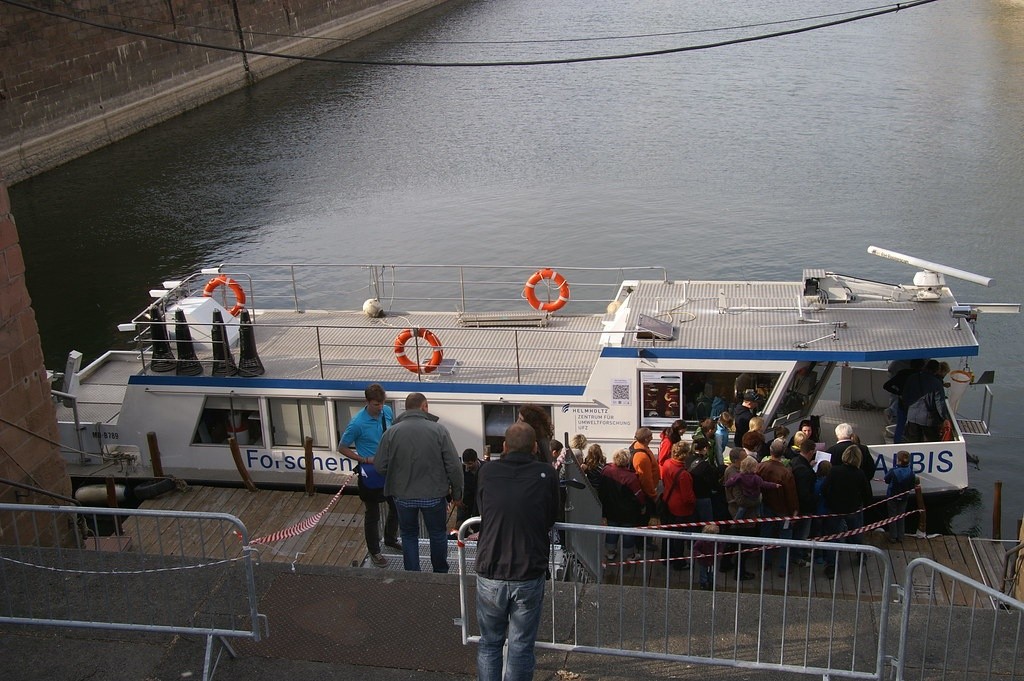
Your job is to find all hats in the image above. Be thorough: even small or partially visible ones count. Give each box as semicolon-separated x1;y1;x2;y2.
744;390;760;402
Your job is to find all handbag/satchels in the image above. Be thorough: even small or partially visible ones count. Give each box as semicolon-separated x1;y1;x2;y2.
360;461;387;488
655;494;671;518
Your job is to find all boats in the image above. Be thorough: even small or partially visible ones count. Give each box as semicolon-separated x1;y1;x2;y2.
45;243;1023;500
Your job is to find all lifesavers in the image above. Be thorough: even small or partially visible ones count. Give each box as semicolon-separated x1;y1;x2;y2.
203;276;246;317
524;270;570;312
392;327;444;374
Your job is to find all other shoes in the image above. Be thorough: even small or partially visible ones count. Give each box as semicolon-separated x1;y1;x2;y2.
824;565;834;580
858;552;866;566
888;536;903;543
815;556;824;563
799;560;811;567
627;553;641;562
606;549;617;559
718;560;735;572
639;541;658;551
734;571;755;580
684;563;690;570
800;552;811;558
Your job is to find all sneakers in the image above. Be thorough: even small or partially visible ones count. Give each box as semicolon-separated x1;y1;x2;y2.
372;553;388;567
385;541;404;553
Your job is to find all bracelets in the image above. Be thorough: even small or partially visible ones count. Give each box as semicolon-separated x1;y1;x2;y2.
360;457;369;465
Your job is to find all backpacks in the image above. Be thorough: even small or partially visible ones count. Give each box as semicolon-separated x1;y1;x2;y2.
627;441;651;473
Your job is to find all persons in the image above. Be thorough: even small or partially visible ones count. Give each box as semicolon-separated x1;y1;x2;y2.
450;357;952;588
885;451;919;542
821;444;872;580
372;392;464;573
473;422;559;681
336;384;403;567
